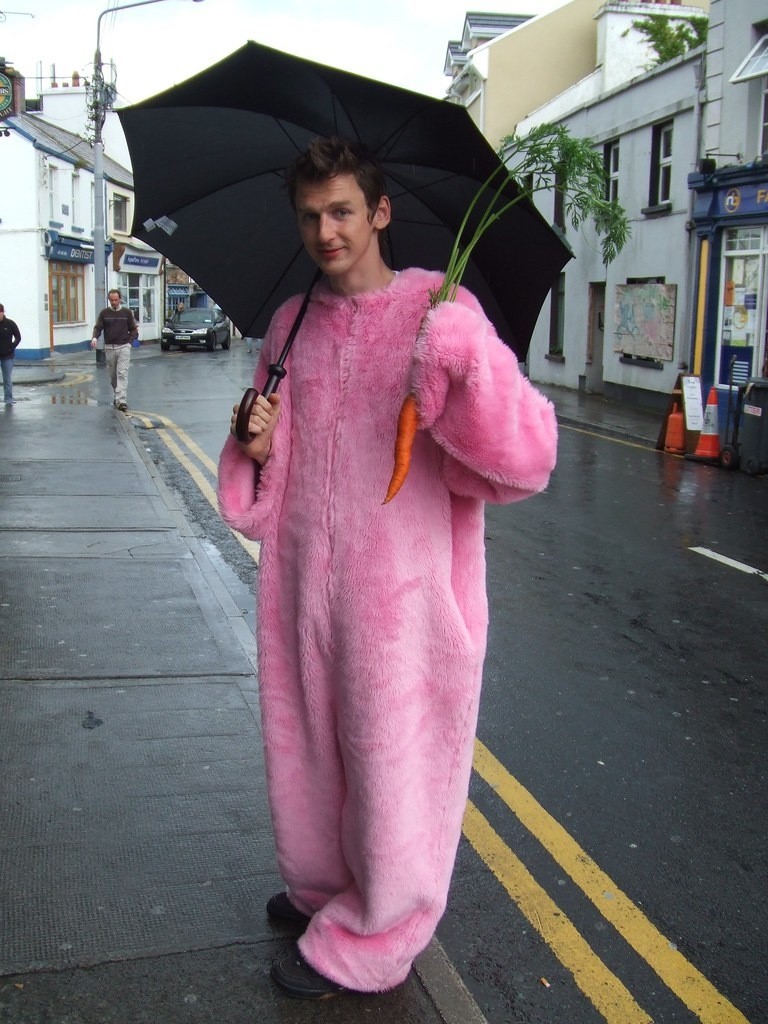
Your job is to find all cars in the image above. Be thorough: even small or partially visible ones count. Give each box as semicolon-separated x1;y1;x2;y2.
160;307;233;351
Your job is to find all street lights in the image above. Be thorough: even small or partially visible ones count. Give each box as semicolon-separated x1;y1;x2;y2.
94;0;204;368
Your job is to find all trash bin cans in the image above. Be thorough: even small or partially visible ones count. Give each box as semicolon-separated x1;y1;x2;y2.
738;378;768;477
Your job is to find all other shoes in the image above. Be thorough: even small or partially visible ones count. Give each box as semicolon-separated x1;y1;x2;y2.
118;403;127;410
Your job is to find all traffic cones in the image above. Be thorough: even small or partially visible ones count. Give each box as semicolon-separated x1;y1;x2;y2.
684;385;723;464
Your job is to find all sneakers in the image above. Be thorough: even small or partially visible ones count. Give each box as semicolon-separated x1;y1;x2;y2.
266;892;296;917
271;945;356;1000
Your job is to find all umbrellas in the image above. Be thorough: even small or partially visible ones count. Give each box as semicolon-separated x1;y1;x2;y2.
112;39;576;448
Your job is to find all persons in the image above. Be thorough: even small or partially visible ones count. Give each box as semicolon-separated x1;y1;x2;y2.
246;337;261;353
175;302;185;313
216;129;558;996
90;289;138;410
0;304;21;406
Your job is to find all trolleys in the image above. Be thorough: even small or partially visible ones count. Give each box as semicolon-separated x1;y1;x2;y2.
719;355;768;471
